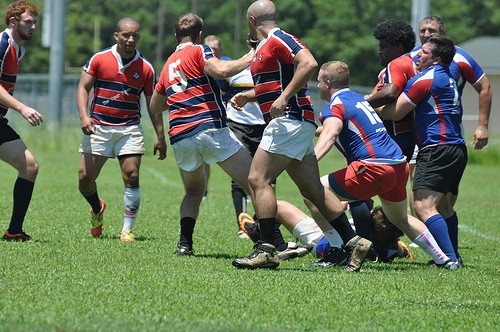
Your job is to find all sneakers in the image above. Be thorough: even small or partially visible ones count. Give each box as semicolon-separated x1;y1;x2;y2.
237;212;260;244
177;242;192;255
120;233;136;242
397;241;413;261
89;199;107;236
313;244;351;268
276;241;310;261
4;230;35;242
430;259;455;271
238;231;250;239
232;246;280;268
339;236;373;272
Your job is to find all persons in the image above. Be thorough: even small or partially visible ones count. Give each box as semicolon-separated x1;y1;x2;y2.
373;35;469;272
313;61;459;272
407;15;493;270
224;32;277;239
228;0;375;273
150;12;315;262
75;17;167;244
202;34;232;104
236;199;415;268
0;2;44;243
347;17;419;264
